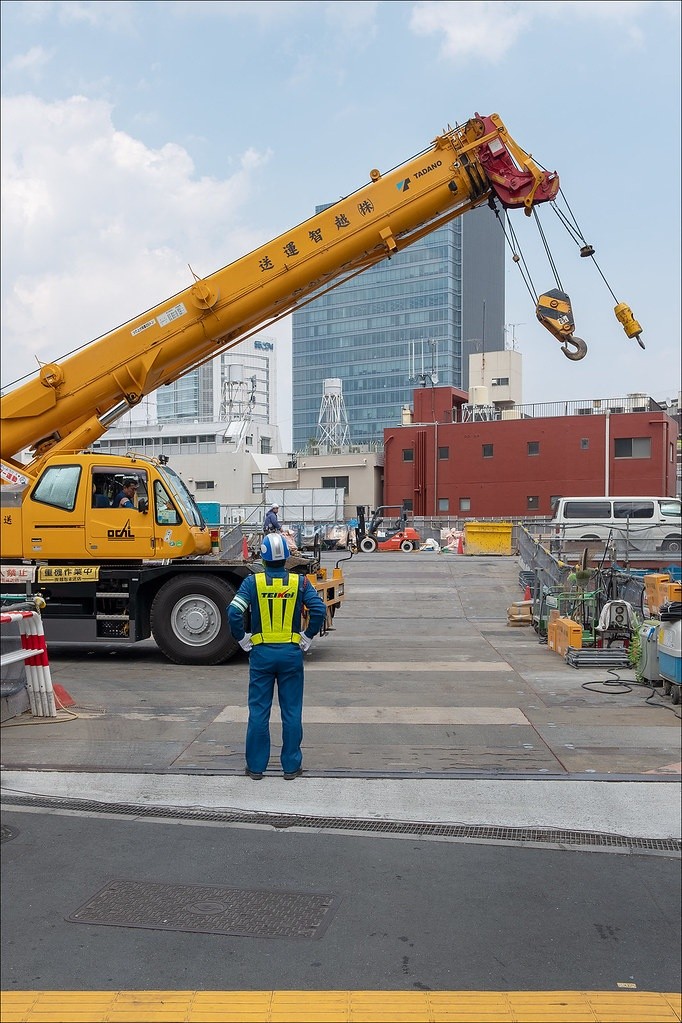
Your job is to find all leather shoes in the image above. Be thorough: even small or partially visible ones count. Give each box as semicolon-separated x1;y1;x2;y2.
245;764;263;780
284;765;302;779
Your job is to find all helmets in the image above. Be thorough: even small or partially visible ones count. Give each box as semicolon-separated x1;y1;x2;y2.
260;533;291;561
271;503;278;508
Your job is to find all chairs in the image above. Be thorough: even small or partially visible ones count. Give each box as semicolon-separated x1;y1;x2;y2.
93;479;112;509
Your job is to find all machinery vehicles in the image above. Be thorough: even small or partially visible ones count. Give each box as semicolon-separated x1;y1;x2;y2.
360;505;420;552
0;109;645;665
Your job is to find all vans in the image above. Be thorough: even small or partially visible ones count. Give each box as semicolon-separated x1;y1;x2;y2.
551;497;682;553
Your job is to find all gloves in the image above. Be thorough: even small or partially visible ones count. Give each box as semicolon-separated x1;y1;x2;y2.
299;631;311;651
239;631;253;650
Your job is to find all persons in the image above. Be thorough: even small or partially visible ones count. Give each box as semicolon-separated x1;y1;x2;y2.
109;478;138;508
226;533;328;780
263;503;283;535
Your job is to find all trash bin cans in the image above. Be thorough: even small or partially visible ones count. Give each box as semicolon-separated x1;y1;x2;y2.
639;619;664;686
464;522;512;555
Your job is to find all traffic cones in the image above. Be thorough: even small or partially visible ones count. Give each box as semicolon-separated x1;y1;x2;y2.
456;537;464;555
524;585;533;601
